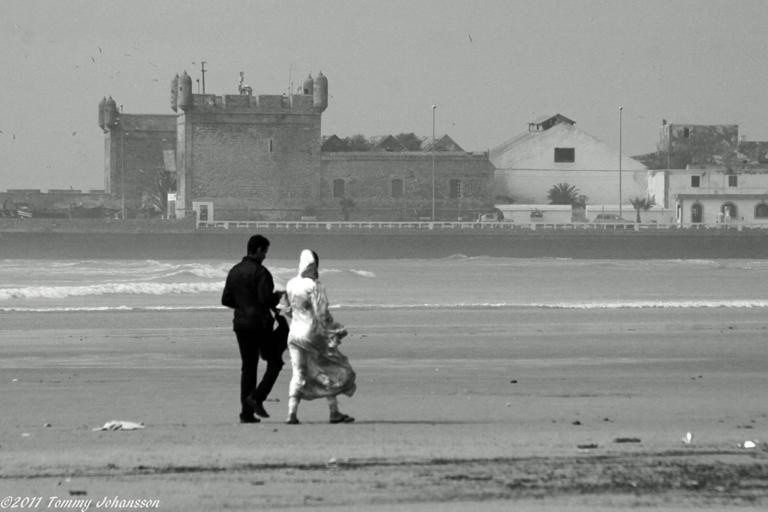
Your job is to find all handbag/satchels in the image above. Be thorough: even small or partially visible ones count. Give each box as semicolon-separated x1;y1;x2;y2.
257;317;289;360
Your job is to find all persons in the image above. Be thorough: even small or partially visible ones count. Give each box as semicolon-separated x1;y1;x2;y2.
286;246;356;425
222;234;285;422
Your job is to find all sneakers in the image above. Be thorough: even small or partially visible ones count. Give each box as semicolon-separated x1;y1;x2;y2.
329;413;353;423
287;415;298;424
246;395;268;416
240;412;259;422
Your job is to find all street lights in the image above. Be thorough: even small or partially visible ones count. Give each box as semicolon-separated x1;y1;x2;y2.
429;102;438;222
617;104;623;219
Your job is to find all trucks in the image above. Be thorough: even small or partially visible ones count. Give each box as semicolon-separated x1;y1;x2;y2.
584;204;677;229
477;198;575;230
